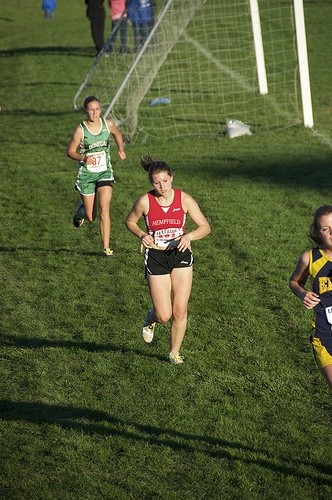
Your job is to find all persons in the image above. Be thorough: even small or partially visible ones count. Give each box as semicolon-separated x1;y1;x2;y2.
289;205;332;388
126;161;211;363
68;97;126;255
126;0;155;53
85;0;106;56
106;0;131;53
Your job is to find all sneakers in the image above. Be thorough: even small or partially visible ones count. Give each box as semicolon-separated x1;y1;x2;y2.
169;352;185;365
73;204;84;227
102;248;114;256
142;310;156;343
